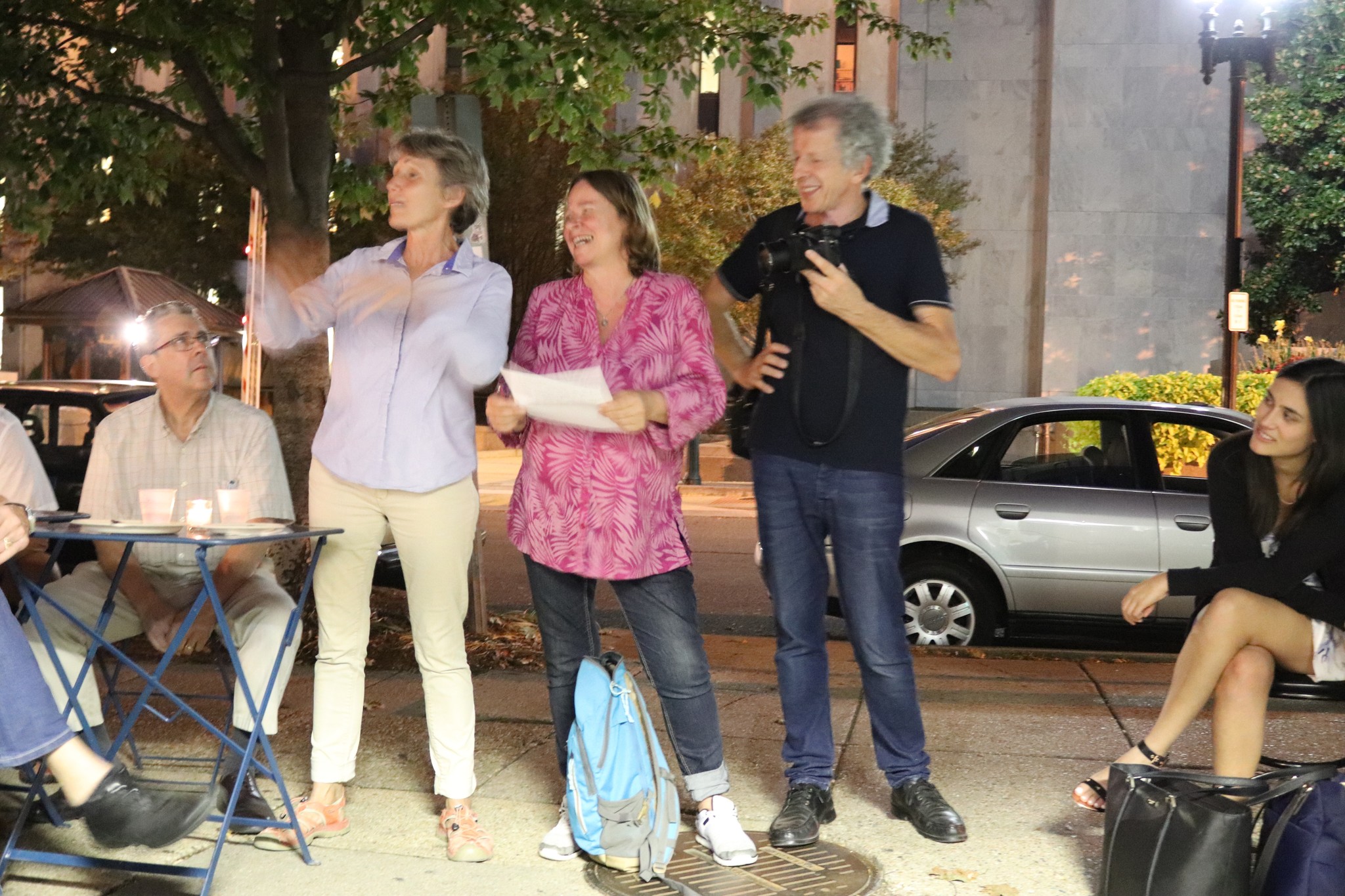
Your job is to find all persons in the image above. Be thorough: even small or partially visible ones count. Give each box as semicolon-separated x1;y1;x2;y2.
702;94;969;845
0;301;303;850
486;168;758;869
1071;358;1345;814
232;123;497;865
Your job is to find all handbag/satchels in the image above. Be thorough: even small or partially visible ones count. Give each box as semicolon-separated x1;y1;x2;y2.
727;382;760;460
1099;762;1338;896
1253;769;1345;896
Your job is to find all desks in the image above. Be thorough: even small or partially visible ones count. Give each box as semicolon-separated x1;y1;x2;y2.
0;521;344;896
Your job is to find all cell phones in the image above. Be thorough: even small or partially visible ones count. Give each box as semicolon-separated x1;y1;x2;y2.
35;512;90;522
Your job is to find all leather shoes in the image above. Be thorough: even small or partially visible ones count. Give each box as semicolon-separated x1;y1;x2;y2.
890;777;968;843
216;768;278;836
769;785;836;847
83;762;216;848
18;783;85;824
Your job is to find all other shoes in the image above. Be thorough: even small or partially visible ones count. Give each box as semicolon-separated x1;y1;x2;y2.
14;756;58;783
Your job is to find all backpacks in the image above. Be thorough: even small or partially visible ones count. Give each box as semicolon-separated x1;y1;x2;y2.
563;650;703;896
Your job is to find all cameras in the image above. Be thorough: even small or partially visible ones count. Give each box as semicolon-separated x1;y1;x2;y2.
757;224;842;279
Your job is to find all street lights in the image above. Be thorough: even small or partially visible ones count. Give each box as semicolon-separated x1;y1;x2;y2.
1195;2;1288;407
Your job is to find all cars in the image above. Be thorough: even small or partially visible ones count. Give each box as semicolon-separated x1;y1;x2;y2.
2;380;157;613
752;396;1321;648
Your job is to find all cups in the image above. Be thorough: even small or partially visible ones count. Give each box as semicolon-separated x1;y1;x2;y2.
138;489;175;524
216;489;250;524
186;498;212;532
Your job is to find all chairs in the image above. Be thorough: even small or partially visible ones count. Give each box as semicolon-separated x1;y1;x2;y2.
1259;665;1345;770
95;632;234;794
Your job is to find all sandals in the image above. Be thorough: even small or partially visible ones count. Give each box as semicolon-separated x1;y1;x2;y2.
1072;739;1171;813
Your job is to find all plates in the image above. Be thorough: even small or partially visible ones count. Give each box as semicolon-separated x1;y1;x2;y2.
203;523;287;536
69;519;187;535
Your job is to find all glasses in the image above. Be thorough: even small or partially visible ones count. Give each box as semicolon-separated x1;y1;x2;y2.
149;333;222;353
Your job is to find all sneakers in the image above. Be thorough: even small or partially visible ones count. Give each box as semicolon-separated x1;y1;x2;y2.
253;784;350;851
437;804;493;863
538;793;583;860
694;795;759;867
